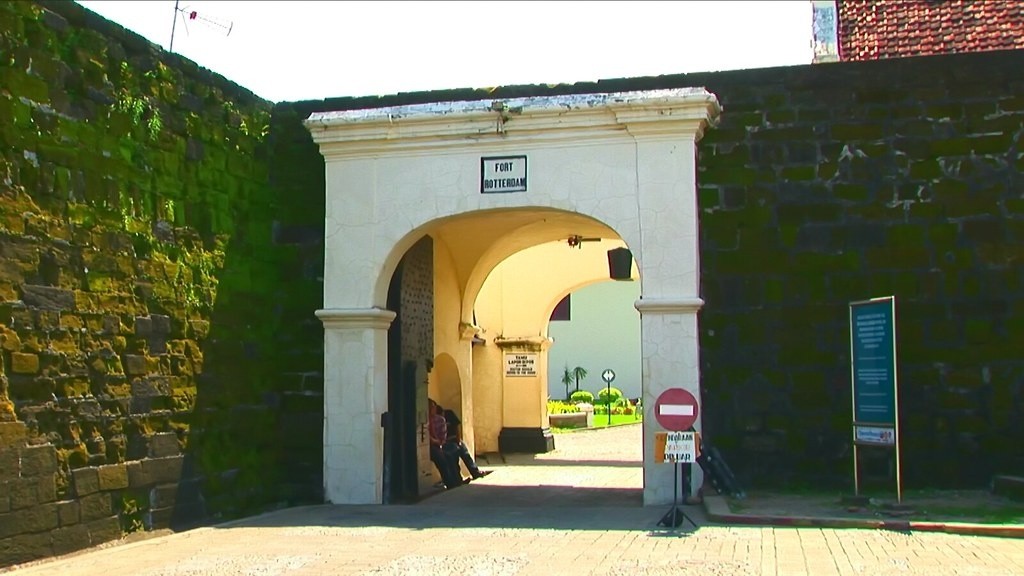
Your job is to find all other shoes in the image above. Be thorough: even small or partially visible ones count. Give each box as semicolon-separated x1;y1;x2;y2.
464;477;471;484
473;471;486;479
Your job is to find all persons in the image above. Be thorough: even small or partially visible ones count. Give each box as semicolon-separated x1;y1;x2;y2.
428;398;493;489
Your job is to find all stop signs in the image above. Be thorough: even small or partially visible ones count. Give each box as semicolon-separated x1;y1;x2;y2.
654;388;698;431
